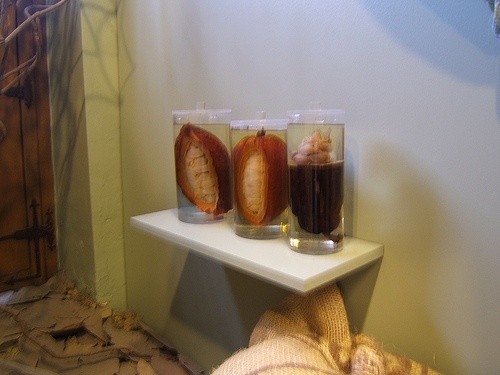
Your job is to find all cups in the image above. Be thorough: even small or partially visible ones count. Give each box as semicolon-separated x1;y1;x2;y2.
172;110;233;224
287;110;345;255
229;119;290;240
